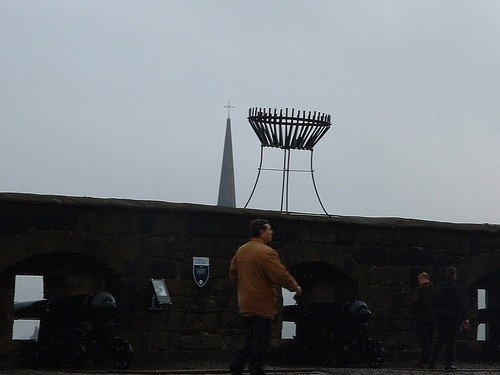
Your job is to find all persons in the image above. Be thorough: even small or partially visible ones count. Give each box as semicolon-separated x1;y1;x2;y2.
228;219;302;375
411;272;445;370
433;266;470;369
